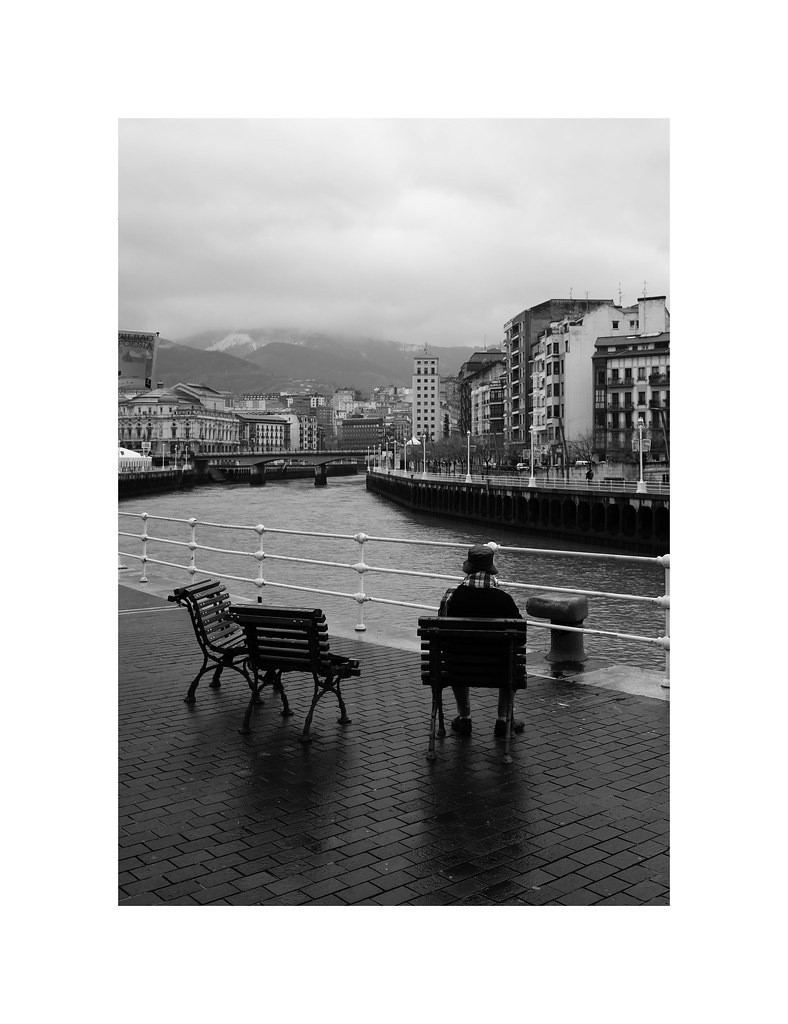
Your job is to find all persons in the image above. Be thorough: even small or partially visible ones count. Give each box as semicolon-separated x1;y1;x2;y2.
437;544;525;734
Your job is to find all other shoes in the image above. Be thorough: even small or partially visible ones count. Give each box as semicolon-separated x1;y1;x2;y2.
494;718;525;735
451;716;472;732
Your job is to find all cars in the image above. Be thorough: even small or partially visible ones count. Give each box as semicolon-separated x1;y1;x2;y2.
515;463;530;471
575;461;592;469
491;462;502;469
482;461;493;469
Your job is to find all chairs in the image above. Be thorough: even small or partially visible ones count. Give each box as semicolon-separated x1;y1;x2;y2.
229;603;361;734
417;616;527;764
167;579;266;704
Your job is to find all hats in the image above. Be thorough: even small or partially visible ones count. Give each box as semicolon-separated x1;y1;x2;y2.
462;545;498;574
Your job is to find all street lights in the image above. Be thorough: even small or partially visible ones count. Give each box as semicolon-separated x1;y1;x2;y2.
367;437;407;478
636;416;649;494
422;434;428;479
175;445;178;470
186;446;188;470
162;444;166;472
465;430;473;483
527;424;538;488
